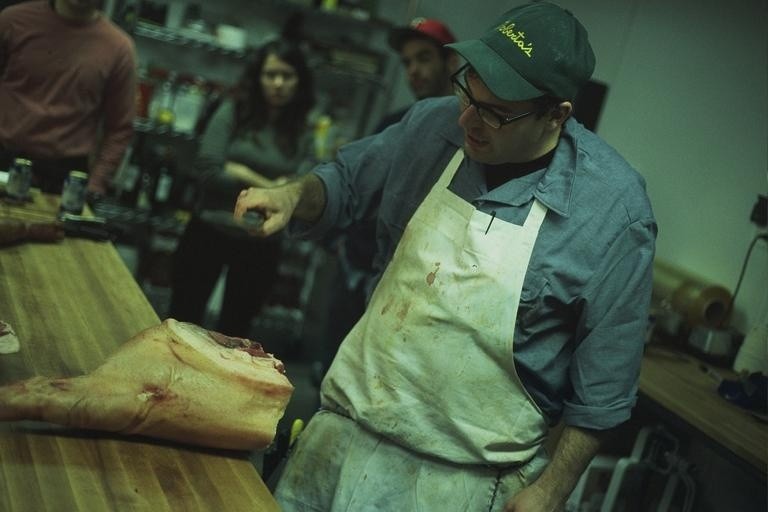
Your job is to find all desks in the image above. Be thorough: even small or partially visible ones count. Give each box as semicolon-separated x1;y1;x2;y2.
566;337;768;512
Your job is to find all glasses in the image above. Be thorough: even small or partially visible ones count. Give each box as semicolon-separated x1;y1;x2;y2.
450;61;546;129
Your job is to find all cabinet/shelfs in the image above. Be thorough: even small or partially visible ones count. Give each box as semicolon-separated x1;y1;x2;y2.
99;0;404;339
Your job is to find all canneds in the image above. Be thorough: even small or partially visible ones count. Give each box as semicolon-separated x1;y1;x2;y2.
5;159;32;204
57;171;88;222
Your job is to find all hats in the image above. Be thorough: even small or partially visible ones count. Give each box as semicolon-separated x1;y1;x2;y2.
444;2;596;103
388;18;455;53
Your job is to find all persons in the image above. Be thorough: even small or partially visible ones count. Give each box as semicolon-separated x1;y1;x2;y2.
312;14;464;379
0;1;142;203
165;38;317;336
227;3;662;510
